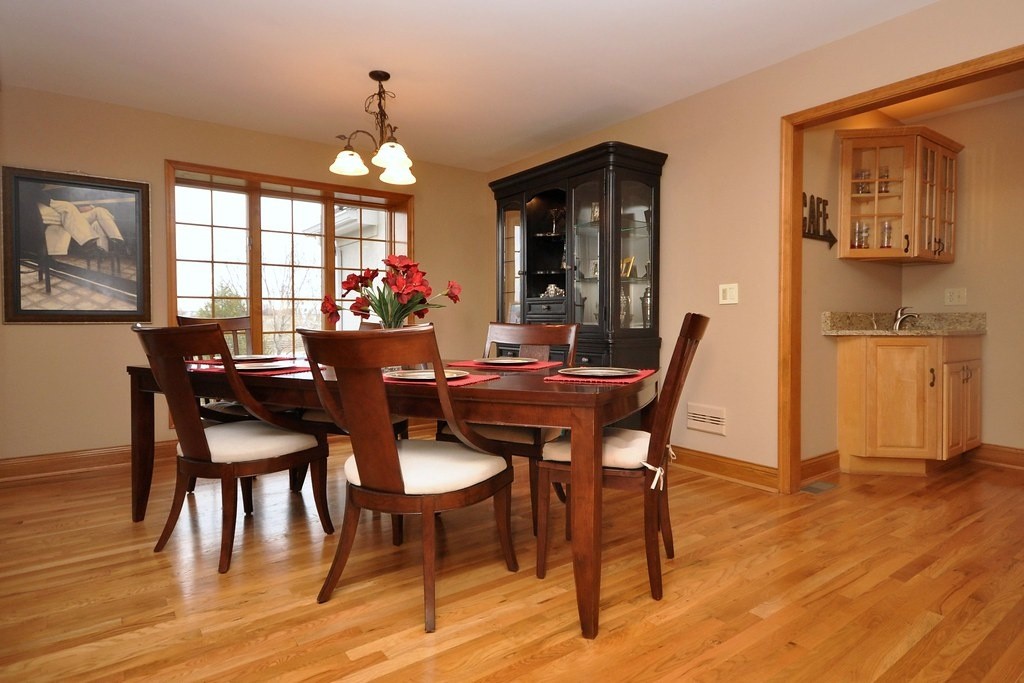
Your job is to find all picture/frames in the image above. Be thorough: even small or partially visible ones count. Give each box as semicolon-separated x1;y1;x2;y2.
1;166;153;326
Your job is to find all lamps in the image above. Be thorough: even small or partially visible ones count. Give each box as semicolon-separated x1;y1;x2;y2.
329;70;416;185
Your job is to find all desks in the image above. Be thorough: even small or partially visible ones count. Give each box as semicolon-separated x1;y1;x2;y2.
127;359;663;640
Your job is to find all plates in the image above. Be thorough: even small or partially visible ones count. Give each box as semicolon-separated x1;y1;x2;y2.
473;357;539;363
382;370;470;379
214;355;274;360
557;367;640;376
215;362;294;370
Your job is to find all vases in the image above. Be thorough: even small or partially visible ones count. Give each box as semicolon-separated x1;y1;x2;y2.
380;321;404;371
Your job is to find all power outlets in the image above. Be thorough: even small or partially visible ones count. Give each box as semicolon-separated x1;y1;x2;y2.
946;287;966;306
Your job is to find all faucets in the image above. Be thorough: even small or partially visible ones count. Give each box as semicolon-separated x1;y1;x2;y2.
893;305;919;332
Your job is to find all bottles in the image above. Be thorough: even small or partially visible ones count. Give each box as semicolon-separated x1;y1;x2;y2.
856;169;871;193
880;221;892;248
852;221;869;249
879;166;889;193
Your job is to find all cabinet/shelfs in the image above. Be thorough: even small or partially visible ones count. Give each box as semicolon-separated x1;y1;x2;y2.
488;142;669;372
836;127;966;264
837;336;983;476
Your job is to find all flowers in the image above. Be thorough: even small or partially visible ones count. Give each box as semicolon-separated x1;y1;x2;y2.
320;255;463;325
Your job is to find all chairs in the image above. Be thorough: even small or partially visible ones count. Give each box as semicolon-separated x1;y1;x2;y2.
302;327;519;633
534;312;708;601
296;323;430;492
434;322;580;536
132;321;335;573
176;316;305;516
